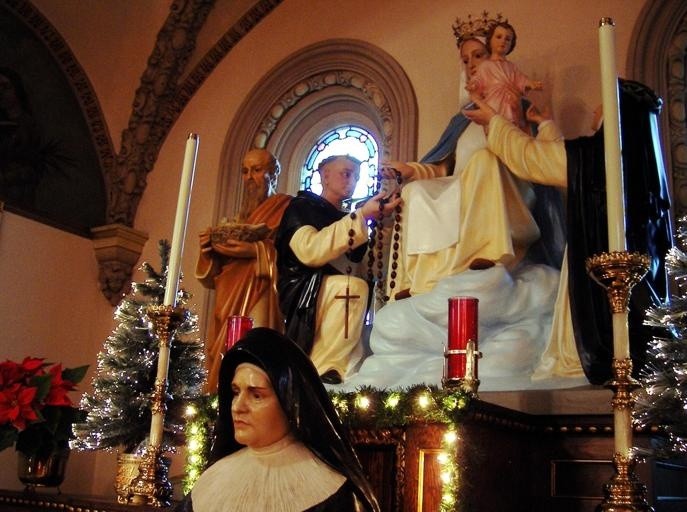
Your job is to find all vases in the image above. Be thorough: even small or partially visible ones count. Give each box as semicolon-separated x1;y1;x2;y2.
17;436;70;495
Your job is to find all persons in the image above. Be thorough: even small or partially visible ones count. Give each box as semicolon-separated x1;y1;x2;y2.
274;153;402;383
372;31;569;301
168;324;380;512
462;76;674;384
193;149;294;396
465;20;545;131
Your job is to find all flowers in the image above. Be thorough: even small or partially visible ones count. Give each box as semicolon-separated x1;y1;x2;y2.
0;354;89;435
